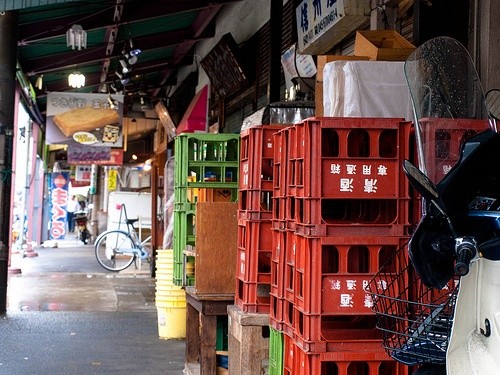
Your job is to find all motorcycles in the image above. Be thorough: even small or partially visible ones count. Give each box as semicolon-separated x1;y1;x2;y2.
364;37;500;375
73;204;94;245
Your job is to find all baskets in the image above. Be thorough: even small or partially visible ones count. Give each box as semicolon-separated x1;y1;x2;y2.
364;242;460;365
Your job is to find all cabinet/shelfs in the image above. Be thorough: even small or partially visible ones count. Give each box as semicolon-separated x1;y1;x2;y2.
131;215;151;270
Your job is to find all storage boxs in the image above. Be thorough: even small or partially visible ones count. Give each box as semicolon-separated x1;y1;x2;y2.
267;114;500;375
314;28;417;117
171;205;197;285
172;131;239;207
233;122;278;219
228;211;272;313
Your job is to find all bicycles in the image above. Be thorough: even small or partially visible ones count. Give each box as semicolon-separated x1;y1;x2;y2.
94;218;152;272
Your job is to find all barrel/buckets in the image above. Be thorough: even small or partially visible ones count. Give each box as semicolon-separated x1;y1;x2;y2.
155;249;190;340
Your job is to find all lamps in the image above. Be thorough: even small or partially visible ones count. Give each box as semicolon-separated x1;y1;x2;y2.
110;80;123;91
118;58;134;73
126;37;141;56
121;48;140;65
114;70;129;84
66;69;87;88
66;24;89;51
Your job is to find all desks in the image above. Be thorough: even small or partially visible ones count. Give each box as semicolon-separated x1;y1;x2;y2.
225;304;271;375
183;292;235;375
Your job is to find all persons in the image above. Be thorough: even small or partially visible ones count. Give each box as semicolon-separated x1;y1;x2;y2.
67;195;78;233
